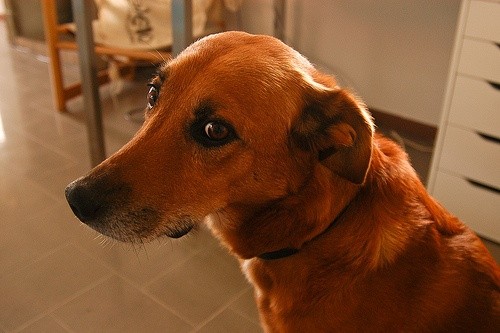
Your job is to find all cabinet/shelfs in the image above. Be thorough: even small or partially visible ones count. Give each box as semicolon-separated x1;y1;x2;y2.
422;1;499;243
40;0;228;113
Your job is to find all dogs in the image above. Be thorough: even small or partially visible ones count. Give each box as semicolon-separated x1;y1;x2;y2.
64;30;500;332
